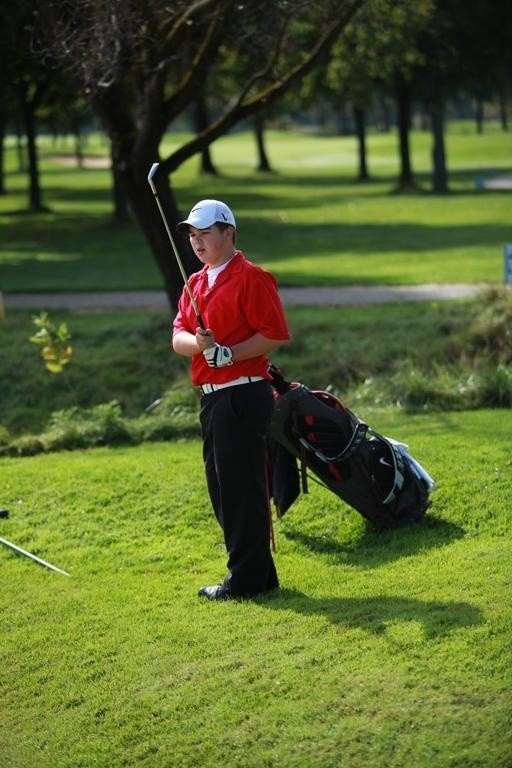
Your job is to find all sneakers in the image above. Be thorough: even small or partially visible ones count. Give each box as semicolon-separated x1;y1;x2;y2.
198;584;280;599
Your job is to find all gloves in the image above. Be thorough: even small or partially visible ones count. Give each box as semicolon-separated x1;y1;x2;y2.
203;342;233;368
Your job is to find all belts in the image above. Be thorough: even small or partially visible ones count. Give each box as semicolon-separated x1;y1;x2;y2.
201;375;265;395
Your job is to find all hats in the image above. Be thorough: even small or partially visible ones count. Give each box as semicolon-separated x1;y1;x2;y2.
176;198;236;231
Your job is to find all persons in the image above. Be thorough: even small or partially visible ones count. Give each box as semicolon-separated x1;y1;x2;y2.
170;198;294;601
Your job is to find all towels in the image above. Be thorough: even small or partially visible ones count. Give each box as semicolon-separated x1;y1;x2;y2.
369;432;435;494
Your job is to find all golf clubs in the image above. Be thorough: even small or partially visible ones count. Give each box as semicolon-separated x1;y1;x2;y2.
147;163;209;336
